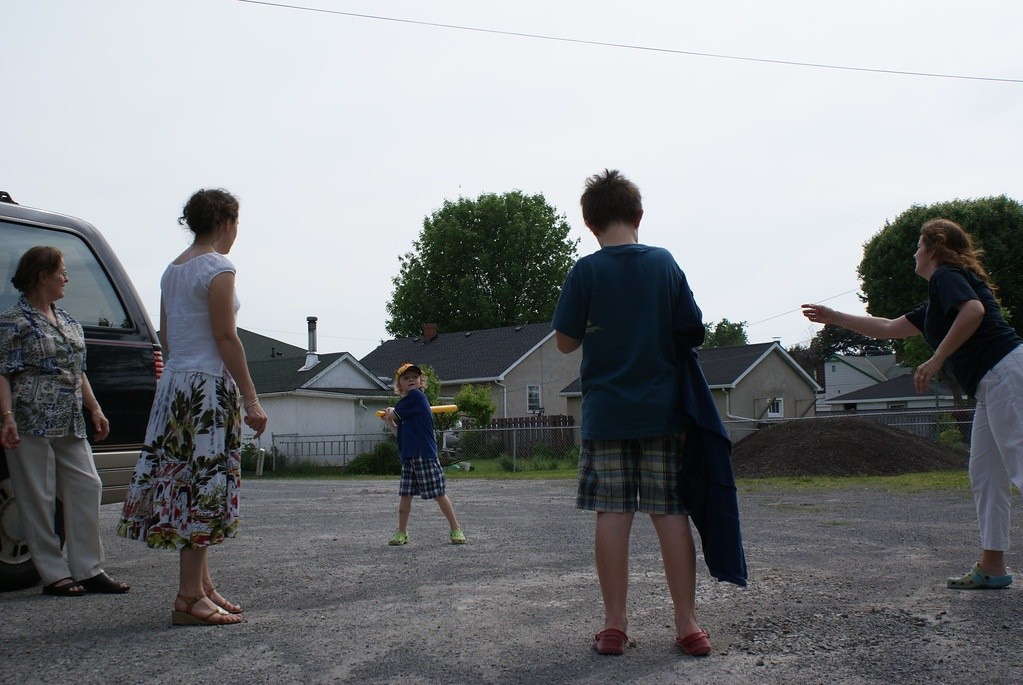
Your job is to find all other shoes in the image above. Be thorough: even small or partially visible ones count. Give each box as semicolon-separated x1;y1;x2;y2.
676;629;712;656
592;629;628;655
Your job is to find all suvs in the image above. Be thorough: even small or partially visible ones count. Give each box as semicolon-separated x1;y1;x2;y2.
0;190;164;592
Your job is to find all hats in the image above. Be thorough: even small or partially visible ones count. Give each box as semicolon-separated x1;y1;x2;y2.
397;364;421;382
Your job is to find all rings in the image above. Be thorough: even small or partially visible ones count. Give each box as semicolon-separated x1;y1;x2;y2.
250;423;255;428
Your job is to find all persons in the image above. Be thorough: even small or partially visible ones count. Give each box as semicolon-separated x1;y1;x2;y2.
376;362;466;546
552;169;748;657
0;246;131;597
115;189;268;626
800;219;1023;590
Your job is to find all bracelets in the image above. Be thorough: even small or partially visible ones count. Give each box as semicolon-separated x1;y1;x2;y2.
1;411;13;417
244;398;258;412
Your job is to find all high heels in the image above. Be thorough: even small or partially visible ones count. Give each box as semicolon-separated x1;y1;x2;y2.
204;588;244;614
171;594;242;625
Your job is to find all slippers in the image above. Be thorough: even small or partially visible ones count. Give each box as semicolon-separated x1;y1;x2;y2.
947;562;1013;589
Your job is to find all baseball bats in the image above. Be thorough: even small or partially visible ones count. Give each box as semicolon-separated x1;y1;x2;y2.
376;405;458;417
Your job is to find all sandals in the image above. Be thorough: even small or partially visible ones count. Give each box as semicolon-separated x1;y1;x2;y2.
389;530;409;544
43;577;87;596
450;528;466;544
78;572;130;593
